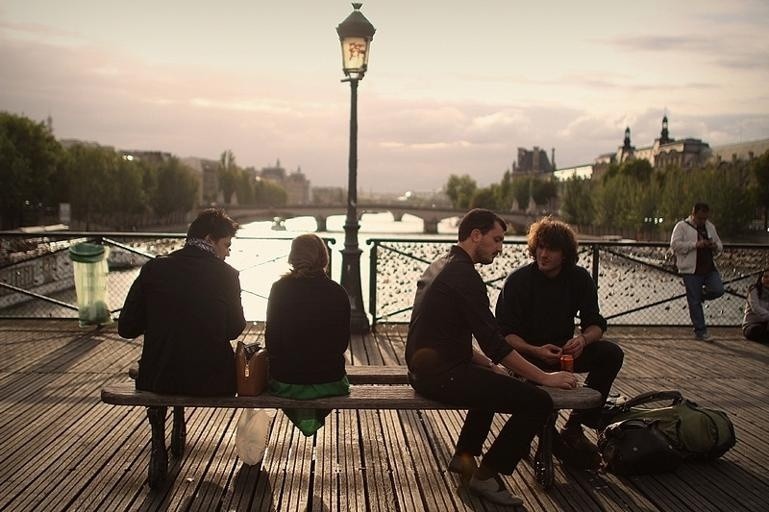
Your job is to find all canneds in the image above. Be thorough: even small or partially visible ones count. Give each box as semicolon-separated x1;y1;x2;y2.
560;354;575;374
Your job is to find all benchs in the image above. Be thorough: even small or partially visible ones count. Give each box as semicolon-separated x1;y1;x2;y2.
131;357;517;455
102;383;603;488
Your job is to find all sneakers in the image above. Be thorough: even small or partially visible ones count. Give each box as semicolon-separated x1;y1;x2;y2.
468;467;523;505
448;454;478;476
702;334;713;341
560;426;597;449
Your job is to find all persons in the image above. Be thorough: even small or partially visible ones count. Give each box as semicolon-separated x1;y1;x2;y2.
741;267;768;344
498;215;624;455
670;202;727;340
265;233;352;438
117;207;246;399
405;208;578;507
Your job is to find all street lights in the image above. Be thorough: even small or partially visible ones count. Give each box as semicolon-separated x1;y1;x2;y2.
333;1;375;339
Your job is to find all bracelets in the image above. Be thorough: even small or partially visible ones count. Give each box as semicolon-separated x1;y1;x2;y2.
580;333;589;350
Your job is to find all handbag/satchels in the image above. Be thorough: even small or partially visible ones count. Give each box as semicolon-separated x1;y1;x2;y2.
235;341;270;396
236;408;278;466
599;390;736;460
597;417;687;474
